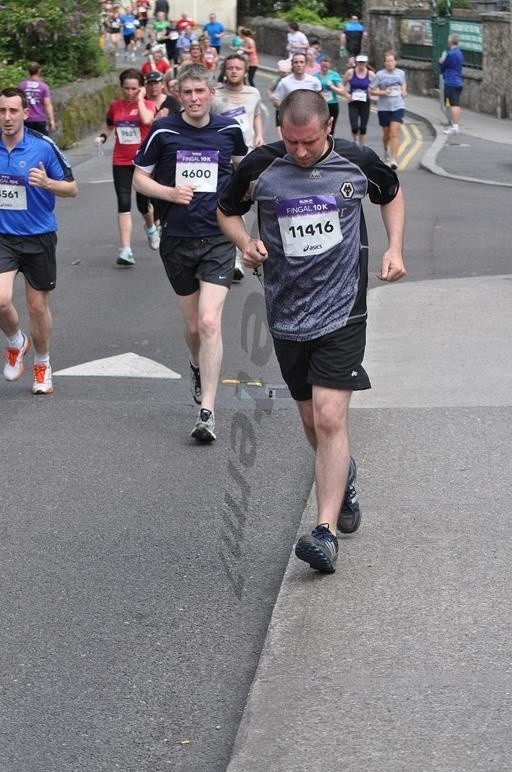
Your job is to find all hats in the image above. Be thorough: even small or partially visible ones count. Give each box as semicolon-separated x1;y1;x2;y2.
356;55;369;63
145;71;162;82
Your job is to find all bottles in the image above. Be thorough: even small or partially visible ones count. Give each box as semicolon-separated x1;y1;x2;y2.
442;51;448;57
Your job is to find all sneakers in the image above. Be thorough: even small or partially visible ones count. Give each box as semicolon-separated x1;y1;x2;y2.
295;525;339;574
190;408;215;442
33;362;52;394
338;458;361;532
190;359;203;404
3;334;30;381
392;162;398;169
233;257;244;280
444;124;459;134
115;247;134;265
384;147;390;161
142;222;160;249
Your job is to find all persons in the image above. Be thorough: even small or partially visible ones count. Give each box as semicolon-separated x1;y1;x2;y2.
438;34;464;134
0;88;79;394
19;62;55;136
217;89;406;575
131;63;249;441
100;2;409;279
